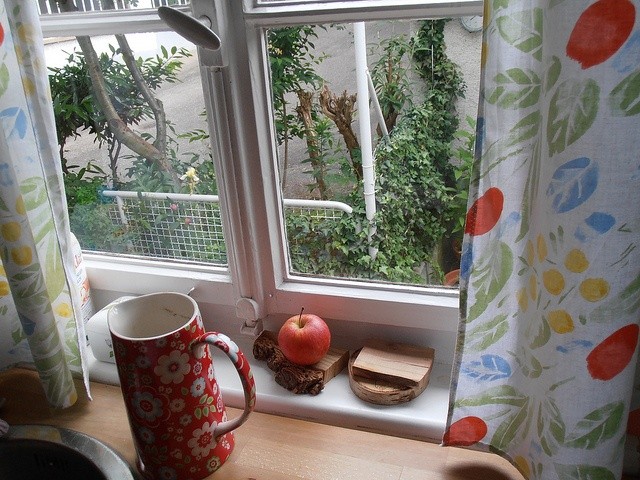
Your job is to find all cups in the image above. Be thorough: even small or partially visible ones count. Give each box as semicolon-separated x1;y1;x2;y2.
107;291;256;480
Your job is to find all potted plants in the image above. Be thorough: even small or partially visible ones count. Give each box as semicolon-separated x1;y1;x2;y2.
448;113;478;258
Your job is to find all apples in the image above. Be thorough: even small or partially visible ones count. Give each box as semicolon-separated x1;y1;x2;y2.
278;303;332;369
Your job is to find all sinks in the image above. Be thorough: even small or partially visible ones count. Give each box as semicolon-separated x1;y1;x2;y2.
0;424;134;480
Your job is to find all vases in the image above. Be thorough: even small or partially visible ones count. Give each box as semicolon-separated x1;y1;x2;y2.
443;269;460;288
